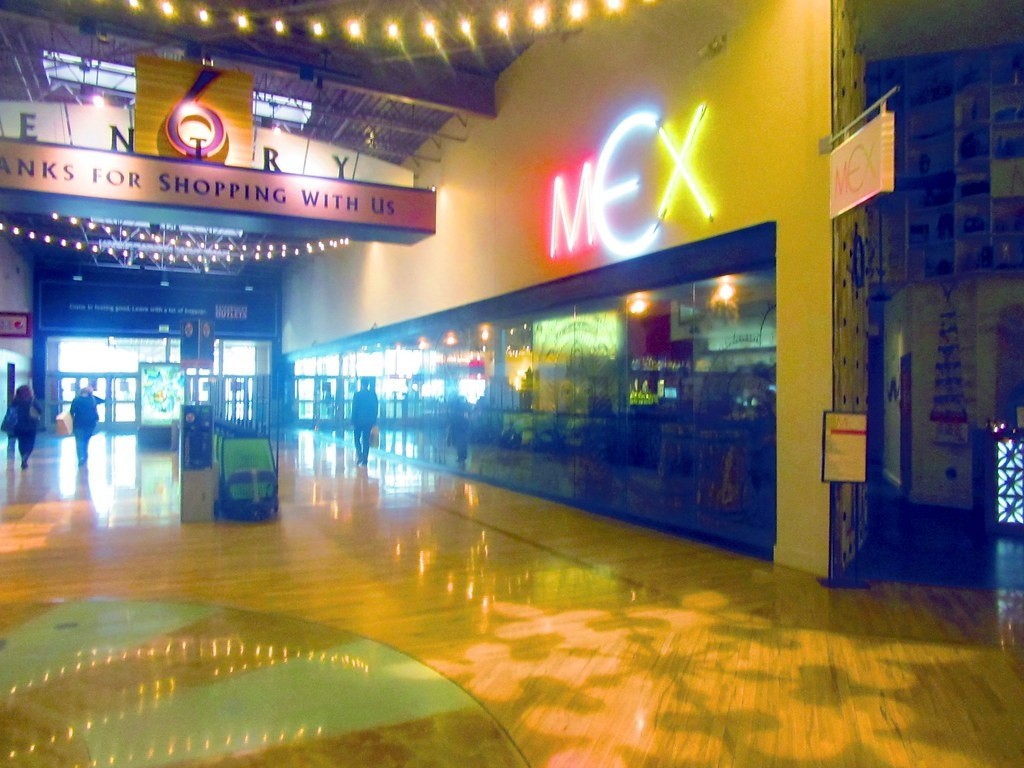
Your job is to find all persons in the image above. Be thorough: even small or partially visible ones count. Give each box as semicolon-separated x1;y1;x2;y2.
70;388;99;465
11;385;43;468
447;391;470;462
352;377;378;465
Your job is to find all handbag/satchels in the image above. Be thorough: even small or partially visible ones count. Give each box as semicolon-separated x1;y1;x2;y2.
56;411;74;436
29;397;40;421
2;399;18;430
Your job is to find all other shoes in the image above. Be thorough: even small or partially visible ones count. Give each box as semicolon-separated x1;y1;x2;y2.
357;454;367;465
22;458;28;469
79;458;85;466
